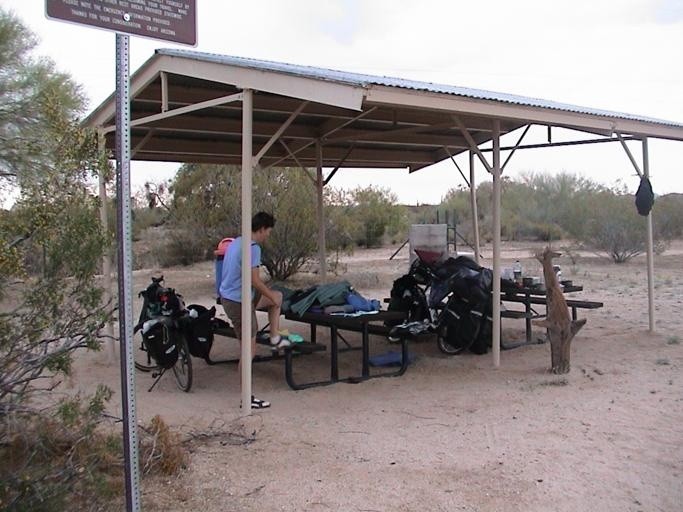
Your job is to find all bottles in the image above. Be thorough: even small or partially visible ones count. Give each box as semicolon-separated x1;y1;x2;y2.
513;260;523;289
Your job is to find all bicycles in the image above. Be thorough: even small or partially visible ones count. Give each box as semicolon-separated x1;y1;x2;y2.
131;275;193;393
385;270;489;355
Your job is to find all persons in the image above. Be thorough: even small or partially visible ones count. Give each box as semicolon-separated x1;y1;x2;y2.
218;211;293;410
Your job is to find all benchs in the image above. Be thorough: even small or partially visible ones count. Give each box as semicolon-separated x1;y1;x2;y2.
382;293;603;351
205;322;328;395
283;313;434;391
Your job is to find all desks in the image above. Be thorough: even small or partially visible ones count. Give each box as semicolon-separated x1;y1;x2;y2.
215;287;389;391
499;282;584;343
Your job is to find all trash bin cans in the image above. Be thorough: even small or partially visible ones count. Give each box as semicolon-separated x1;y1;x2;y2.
215;237;236;304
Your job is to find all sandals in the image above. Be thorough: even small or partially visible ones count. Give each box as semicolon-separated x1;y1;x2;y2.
239;393;271;409
269;333;297;353
